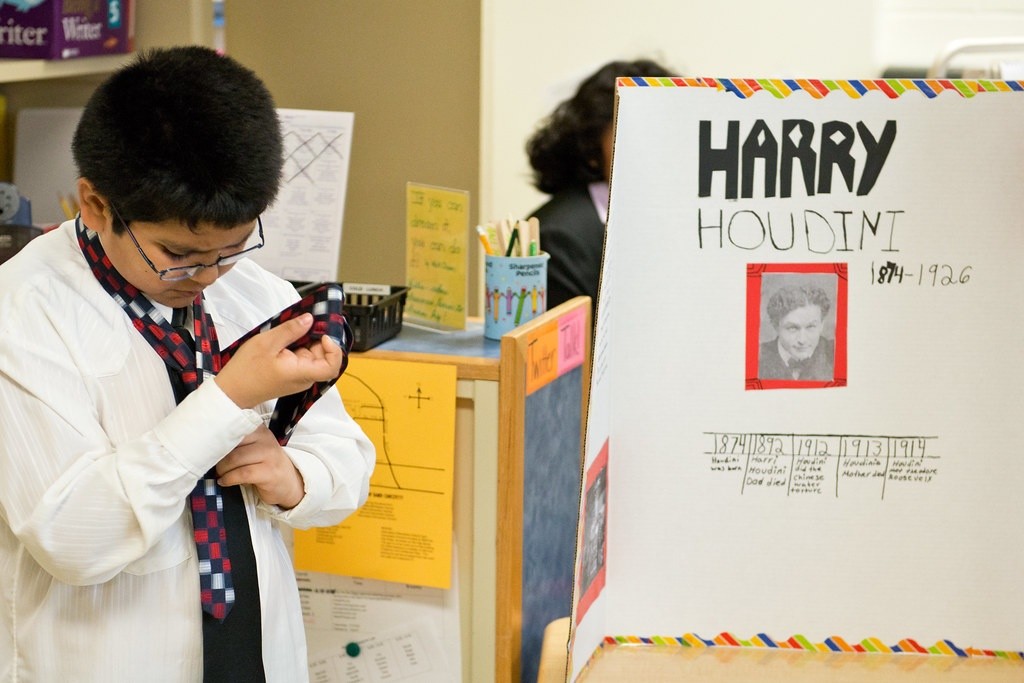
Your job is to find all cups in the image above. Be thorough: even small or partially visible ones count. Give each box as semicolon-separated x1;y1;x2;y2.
484;252;551;340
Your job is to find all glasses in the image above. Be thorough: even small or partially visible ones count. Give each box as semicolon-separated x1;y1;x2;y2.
108;198;264;281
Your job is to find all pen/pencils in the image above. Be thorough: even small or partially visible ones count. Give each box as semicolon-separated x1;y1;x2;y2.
475;216;544;258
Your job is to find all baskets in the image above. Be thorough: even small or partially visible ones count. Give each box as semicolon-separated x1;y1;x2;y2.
295;281;410;352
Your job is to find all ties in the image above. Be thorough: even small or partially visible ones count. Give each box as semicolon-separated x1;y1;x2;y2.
76;208;355;621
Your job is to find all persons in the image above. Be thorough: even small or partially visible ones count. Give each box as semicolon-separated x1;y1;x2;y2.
760;284;834;381
523;59;683;312
0;46;377;683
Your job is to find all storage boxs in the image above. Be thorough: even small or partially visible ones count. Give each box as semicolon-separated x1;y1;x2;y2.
270;278;412;353
